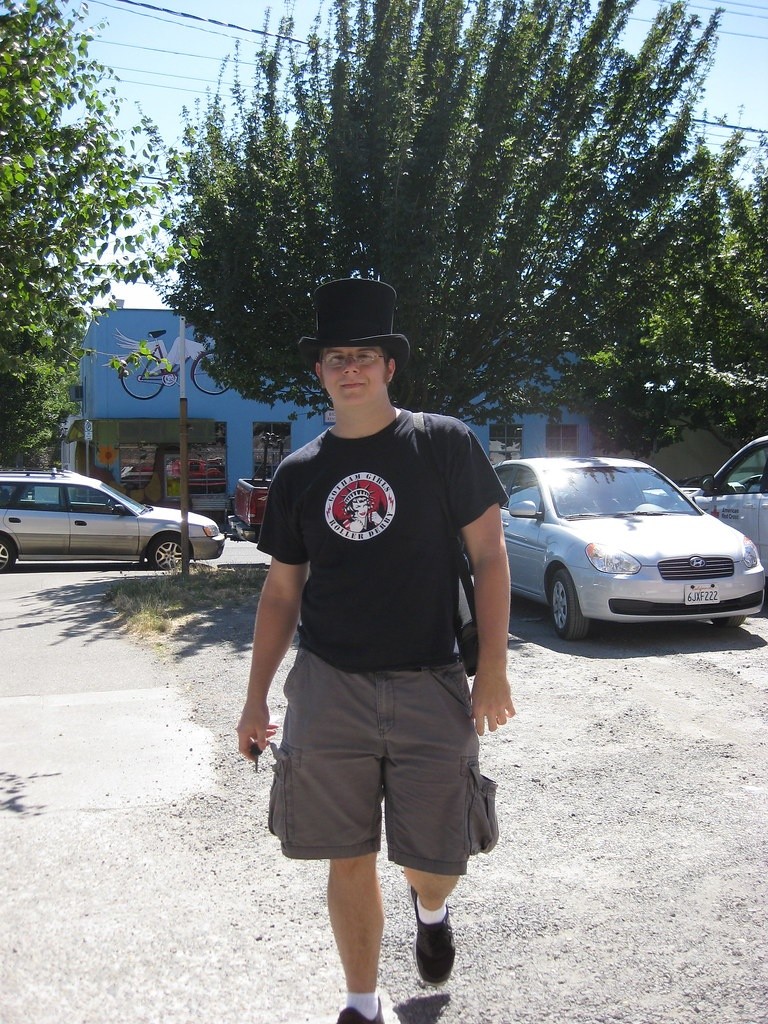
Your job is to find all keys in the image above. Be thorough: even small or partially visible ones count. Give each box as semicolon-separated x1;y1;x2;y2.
250;742;264;773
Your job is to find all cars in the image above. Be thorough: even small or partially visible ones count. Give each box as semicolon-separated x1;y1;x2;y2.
691;434;768;577
493;457;765;640
121;459;226;487
0;468;226;573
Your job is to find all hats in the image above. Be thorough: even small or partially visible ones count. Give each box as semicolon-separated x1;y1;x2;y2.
296;277;410;377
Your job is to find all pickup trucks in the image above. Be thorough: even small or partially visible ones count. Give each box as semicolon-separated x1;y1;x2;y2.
226;434;284;543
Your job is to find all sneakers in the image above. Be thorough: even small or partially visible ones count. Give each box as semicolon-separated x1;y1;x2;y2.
335;996;388;1024
406;881;456;986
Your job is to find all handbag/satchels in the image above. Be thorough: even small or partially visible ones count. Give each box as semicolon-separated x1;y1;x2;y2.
455;570;510;676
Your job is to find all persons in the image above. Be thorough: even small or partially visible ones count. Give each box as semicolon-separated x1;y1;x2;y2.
235;279;515;1024
